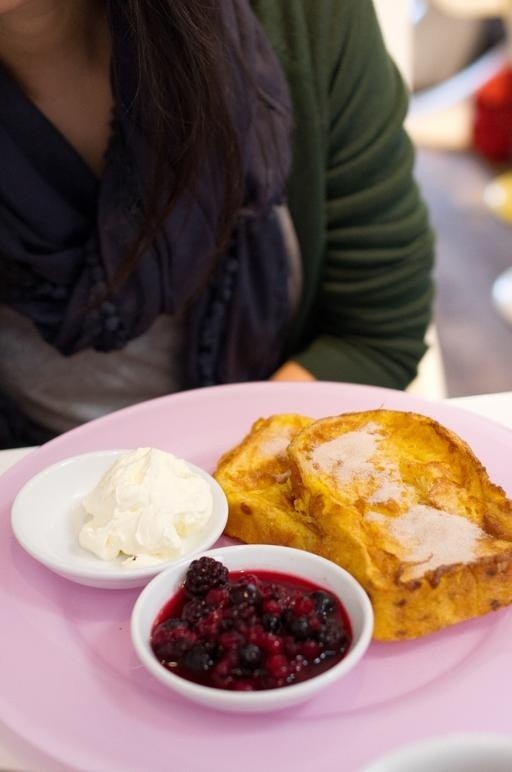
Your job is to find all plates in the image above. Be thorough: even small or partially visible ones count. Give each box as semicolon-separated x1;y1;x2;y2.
9;446;230;591
0;380;512;772
129;544;376;713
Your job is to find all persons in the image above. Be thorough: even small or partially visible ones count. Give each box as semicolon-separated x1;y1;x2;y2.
0;0;441;450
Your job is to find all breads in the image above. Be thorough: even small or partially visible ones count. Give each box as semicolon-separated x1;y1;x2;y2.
212;413;319;554
288;410;512;644
